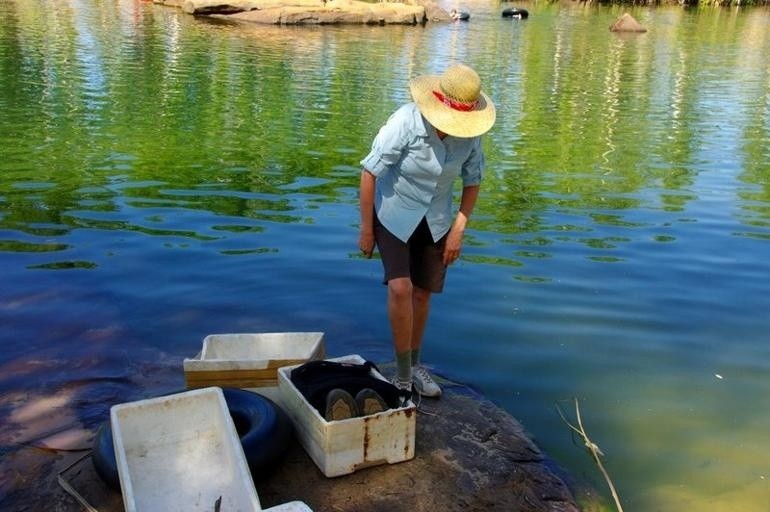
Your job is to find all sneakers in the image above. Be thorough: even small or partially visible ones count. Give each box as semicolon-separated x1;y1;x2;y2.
391;363;441;401
325;387;387;422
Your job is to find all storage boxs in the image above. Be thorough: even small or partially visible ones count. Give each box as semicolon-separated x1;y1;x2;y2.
110;386;262;512
184;332;326;406
278;354;417;478
263;501;314;512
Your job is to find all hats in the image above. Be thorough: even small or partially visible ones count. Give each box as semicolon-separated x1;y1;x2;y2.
410;66;495;139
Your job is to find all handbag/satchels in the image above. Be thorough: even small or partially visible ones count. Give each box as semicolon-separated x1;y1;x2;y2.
292;361;398;418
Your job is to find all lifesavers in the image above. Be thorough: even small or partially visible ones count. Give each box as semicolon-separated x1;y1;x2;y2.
448;12;471;21
502;7;528;21
92;387;291;492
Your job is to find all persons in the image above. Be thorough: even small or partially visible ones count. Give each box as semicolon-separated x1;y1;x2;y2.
451;9;460;19
358;64;496;398
